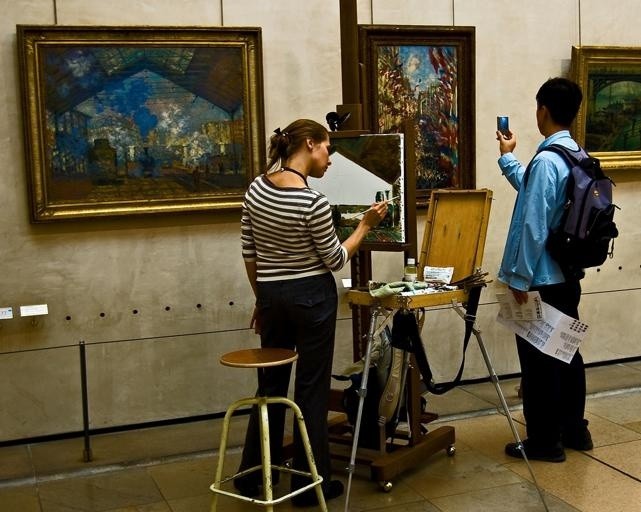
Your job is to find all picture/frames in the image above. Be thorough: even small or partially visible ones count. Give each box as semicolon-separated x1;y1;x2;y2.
304;131;418;251
571;44;640;171
360;23;476;208
15;23;267;225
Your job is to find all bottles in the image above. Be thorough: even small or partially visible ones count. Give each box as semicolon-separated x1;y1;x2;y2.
404;259;418;282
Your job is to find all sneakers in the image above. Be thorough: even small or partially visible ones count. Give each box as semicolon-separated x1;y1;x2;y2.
502;440;567;463
568;433;594;452
227;467;281;489
288;478;344;510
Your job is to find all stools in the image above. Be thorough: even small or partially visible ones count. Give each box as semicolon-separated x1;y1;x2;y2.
209;349;329;512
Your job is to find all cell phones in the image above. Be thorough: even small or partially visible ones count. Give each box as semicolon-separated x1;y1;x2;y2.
497;117;509;136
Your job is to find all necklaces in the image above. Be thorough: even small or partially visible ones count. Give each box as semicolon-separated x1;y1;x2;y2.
282;165;313;190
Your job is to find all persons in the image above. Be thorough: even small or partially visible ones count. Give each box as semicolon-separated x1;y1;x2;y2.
493;78;602;464
236;118;390;508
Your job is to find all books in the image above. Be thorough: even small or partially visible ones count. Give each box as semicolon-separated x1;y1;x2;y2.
495;290;546;322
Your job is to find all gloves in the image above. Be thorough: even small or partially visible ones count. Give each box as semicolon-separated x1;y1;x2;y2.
329;304;413;455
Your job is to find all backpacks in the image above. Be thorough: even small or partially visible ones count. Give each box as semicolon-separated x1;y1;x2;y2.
522;141;620;282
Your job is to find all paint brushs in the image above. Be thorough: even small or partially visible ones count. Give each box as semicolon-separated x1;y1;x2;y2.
449;269;493;289
345;194;400;221
